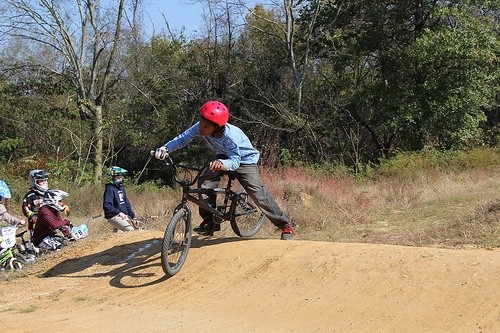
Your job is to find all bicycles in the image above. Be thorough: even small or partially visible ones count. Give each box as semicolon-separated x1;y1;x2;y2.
150;150;268;277
110;214;158;232
0;211;102;272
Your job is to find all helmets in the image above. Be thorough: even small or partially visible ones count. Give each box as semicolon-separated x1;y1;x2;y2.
27;169;49;192
106;166;128;185
0;180;11;199
199;101;229;133
43;189;69;212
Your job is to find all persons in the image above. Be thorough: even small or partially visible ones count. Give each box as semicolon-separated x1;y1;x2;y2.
32;189;73;251
0;180;24;225
102;167;139;231
22;169;70;239
150;101;296;240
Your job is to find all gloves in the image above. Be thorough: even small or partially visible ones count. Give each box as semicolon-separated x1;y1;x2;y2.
155;146;169;160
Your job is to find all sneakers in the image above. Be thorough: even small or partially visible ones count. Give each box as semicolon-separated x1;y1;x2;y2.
193;220;220;232
280;225;296;239
